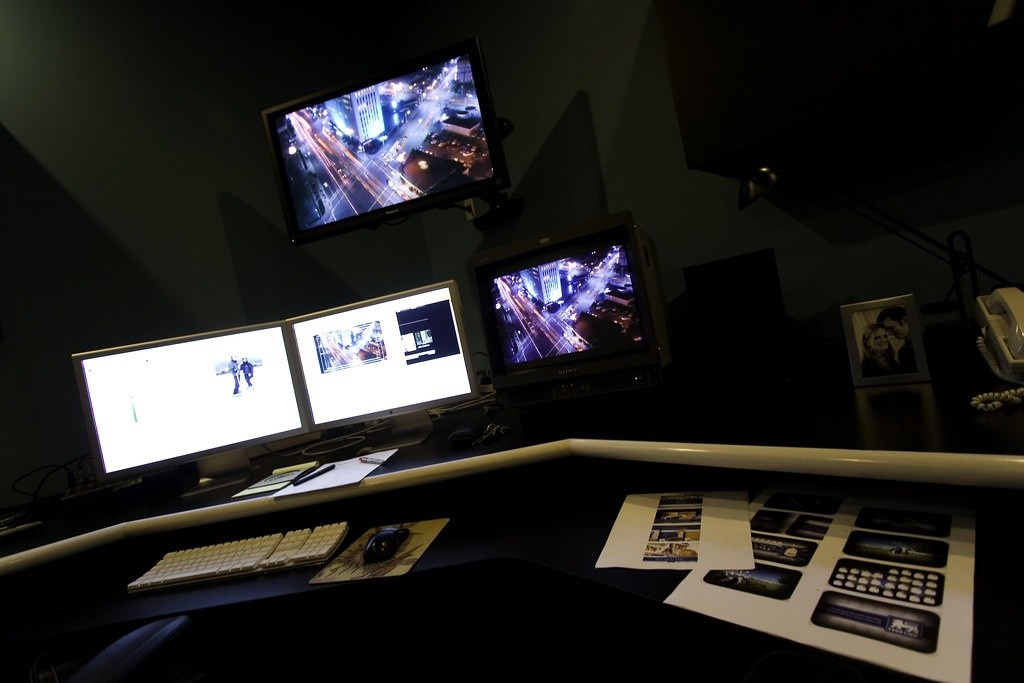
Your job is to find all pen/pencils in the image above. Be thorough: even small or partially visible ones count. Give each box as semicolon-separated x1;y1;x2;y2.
293;464;335;487
360;455;387;465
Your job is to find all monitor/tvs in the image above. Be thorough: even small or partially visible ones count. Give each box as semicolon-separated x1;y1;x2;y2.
261;35;511;247
71;321;310;498
468;210;675;410
285;280;481;454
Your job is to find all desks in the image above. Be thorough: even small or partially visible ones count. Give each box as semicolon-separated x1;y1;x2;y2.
0;322;1024;579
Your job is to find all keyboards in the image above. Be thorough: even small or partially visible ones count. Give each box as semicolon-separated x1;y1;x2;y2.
127;522;350;594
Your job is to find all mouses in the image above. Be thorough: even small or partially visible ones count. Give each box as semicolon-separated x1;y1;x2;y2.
364;527;410;562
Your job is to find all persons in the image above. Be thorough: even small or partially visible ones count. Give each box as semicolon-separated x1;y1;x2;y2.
850;324;895;370
230;355;255;395
876;307;917;373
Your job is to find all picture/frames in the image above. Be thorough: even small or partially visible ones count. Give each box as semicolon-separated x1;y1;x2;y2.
839;294;930;388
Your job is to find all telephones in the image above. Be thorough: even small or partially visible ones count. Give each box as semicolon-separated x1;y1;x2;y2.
971;286;1024;387
